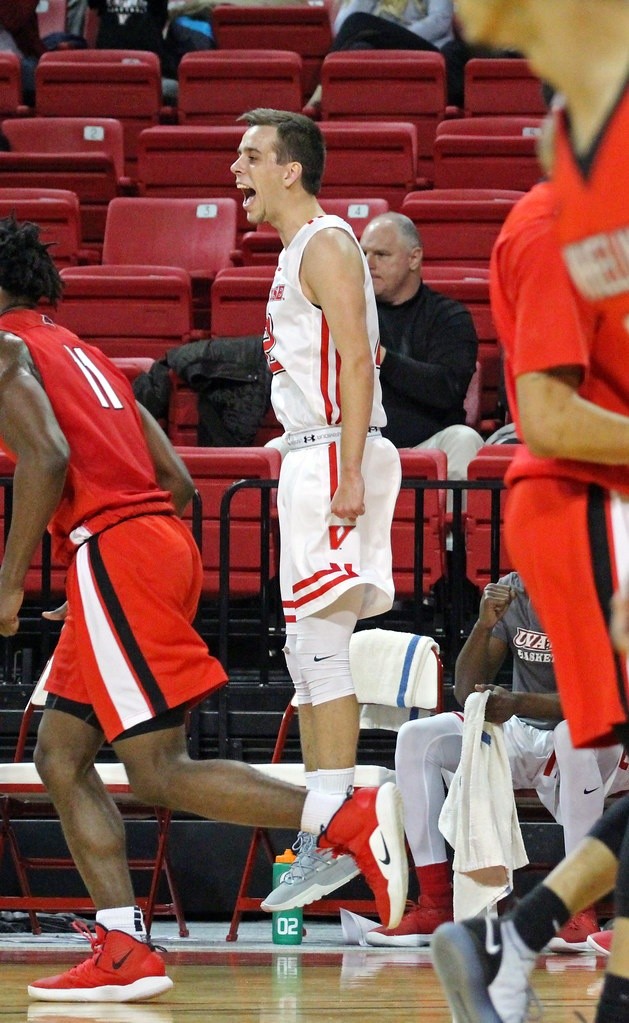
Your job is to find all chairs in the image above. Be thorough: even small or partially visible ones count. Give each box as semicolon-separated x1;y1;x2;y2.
0;0;550;943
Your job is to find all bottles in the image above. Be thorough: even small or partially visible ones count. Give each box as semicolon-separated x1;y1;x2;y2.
272;848;303;945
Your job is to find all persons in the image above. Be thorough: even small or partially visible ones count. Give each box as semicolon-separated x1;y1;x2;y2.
359;211;485;585
430;0;628;1022
0;0;171;115
366;568;564;954
300;0;505;117
230;107;403;913
1;205;408;1001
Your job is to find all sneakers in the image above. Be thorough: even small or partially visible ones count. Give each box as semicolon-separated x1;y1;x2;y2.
430;915;539;1023
586;929;613;953
365;896;457;946
261;831;360;914
548;909;598;951
315;783;408;931
27;922;172;1003
